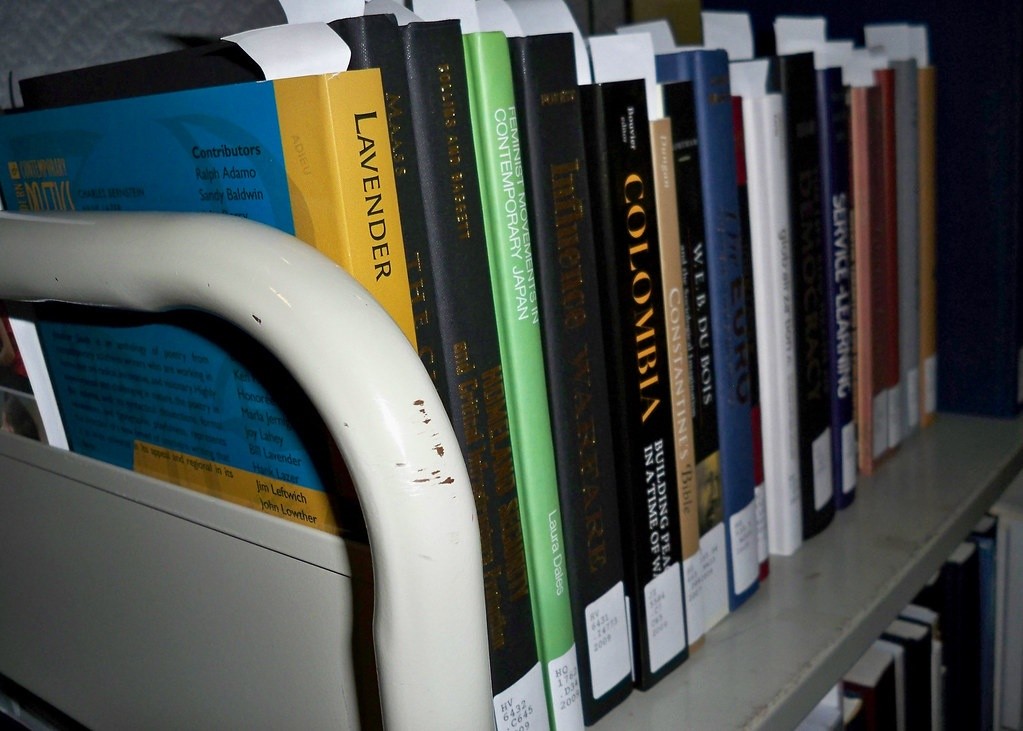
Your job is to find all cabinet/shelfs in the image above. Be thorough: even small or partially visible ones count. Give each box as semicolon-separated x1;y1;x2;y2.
0;211;1022;731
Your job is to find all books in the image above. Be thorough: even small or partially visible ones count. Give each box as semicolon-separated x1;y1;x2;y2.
0;0;1023;731
